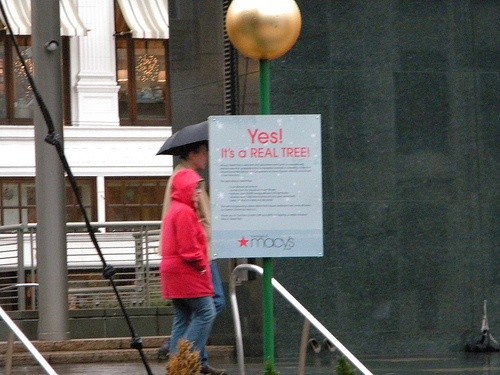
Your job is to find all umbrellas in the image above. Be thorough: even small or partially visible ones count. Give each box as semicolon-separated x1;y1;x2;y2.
156;120;209;156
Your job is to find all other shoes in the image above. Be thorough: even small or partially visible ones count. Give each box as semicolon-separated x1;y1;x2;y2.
199;363;226;375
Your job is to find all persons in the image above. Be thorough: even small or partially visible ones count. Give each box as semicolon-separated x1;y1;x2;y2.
160;170;216;371
163;142;226;375
